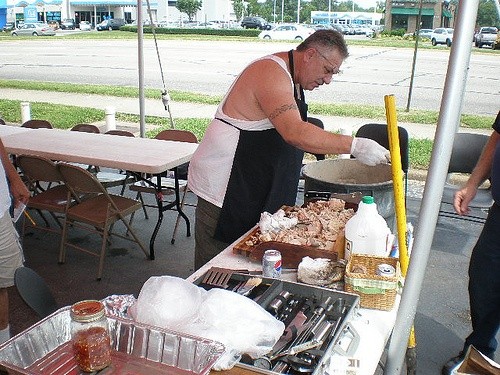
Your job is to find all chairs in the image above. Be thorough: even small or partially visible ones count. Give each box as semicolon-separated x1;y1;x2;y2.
90;130;150;235
14;153;100;266
56;162;153;281
436;133;496;224
349;124;410;212
12;120;62;197
297;116;326;194
46;125;101;191
126;129;203;244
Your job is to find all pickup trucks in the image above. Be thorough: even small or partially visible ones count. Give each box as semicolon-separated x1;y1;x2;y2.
474;26;500;49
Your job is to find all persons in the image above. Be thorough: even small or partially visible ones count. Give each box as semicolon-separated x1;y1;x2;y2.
187;29;392;273
0;140;30;343
442;112;500;375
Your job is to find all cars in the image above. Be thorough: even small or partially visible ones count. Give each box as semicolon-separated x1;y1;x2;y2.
78;21;91;30
60;18;76;30
10;23;56;37
415;28;435;39
431;27;457;47
132;11;381;40
96;18;126;32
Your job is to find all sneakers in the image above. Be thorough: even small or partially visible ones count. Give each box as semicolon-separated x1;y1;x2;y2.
442;350;494;375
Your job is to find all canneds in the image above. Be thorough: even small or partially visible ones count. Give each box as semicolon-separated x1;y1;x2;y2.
70;299;110;371
263;249;281;279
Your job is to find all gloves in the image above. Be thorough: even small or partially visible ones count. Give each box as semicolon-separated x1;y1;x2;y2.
350;136;391;167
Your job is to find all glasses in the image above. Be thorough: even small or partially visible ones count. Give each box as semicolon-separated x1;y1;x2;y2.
312;46;343;77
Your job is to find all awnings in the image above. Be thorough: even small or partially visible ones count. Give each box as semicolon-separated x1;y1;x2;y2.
442;11;453;17
390;7;435;16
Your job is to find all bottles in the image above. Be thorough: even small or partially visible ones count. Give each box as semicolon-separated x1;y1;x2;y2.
345;193;392;275
69;300;112;373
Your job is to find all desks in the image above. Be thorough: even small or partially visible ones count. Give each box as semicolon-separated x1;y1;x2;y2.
0;124;202;261
183;207;417;375
